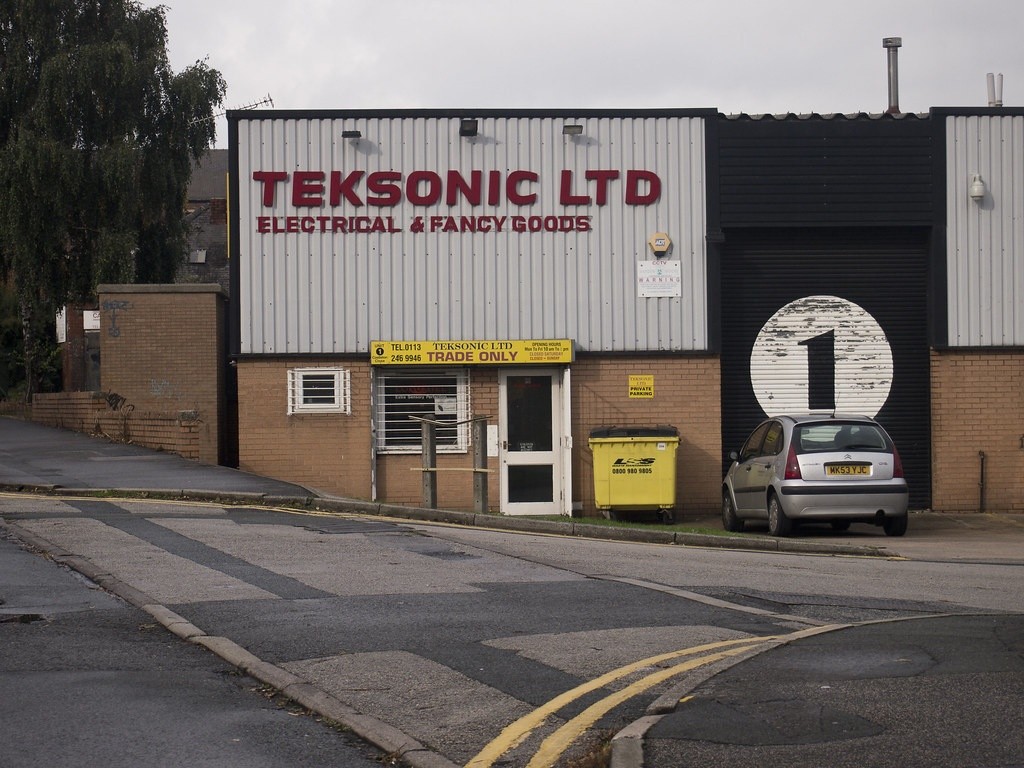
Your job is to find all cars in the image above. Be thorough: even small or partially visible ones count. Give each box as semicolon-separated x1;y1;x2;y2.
720;410;909;536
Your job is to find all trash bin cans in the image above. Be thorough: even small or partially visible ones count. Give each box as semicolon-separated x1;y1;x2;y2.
587;425;681;525
